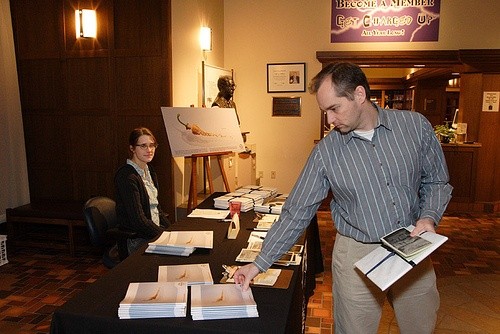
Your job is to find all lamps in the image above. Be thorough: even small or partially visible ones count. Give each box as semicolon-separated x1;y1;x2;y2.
74;8;97;39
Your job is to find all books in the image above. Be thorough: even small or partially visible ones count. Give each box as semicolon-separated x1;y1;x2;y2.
214;186;289;213
354;224;448;290
118;263;259;320
145;231;213;256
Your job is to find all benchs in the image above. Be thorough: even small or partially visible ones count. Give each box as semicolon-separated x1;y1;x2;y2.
6;202;96;252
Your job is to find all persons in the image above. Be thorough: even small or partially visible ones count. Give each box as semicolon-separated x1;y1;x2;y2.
212;75;241;125
234;61;454;334
114;127;169;255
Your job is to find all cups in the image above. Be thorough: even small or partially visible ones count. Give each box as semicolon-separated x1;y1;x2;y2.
457;133;465;143
230;201;242;219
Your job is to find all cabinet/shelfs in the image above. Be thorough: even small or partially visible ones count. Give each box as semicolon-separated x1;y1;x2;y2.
441;142;482;218
370;89;416;111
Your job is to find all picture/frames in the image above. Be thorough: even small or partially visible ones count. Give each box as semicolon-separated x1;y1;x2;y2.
267;63;306;93
272;97;301;117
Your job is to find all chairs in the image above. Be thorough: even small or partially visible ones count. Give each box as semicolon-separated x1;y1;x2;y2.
80;196;126;269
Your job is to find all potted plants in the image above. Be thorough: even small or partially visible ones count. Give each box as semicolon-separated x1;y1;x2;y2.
431;122;456;143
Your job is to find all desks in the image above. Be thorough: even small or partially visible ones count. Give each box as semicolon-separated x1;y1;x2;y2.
48;188;324;334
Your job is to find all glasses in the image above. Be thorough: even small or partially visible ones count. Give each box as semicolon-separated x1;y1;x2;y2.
135;143;159;149
